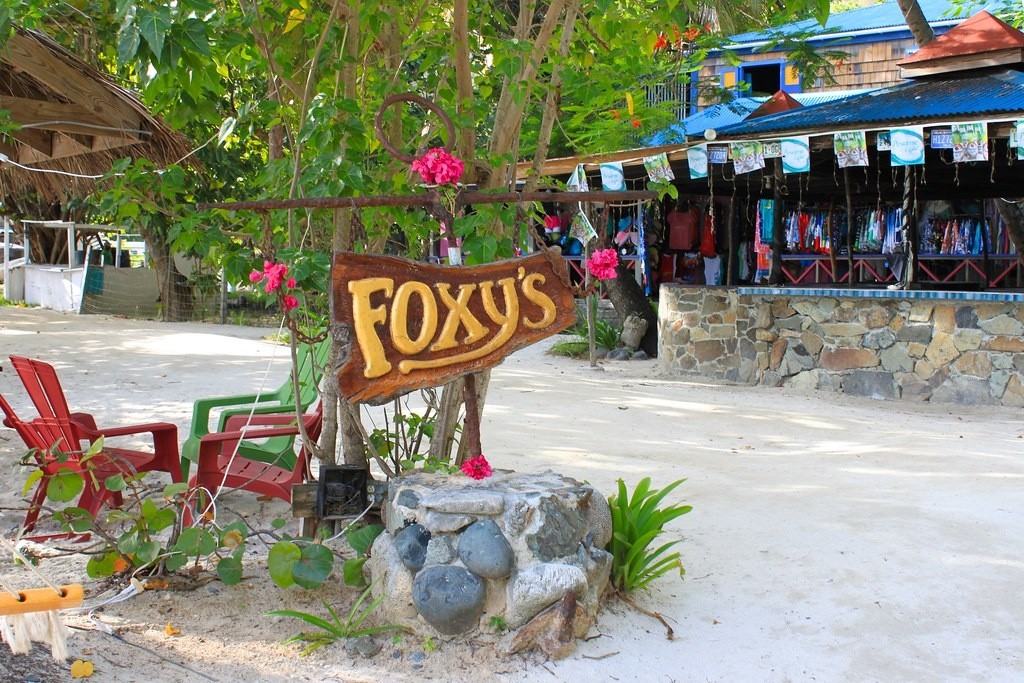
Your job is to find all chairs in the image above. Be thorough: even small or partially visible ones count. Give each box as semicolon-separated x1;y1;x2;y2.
0;355;193;544
187;389;323;541
178;324;331;482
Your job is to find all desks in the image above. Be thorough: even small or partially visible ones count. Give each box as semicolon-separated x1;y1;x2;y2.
781;253;1024;294
563;253;642;301
